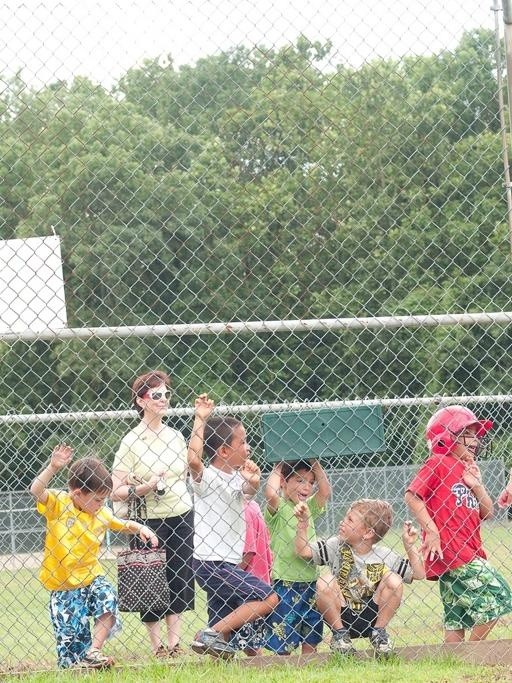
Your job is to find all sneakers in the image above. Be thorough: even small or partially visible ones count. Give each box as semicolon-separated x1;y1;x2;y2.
167;646;186;658
330;630;356;656
192;629;235;659
370;631;394;660
81;649;114;669
153;647;171;661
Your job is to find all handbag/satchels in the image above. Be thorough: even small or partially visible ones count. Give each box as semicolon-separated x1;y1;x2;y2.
117;487;170;612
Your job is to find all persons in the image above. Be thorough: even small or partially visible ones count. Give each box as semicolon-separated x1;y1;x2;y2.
293;498;425;668
264;457;331;655
30;442;159;668
105;371;194;657
404;407;512;643
239;496;274;655
192;397;279;660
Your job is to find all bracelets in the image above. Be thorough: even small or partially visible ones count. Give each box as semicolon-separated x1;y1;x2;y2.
297;522;307;528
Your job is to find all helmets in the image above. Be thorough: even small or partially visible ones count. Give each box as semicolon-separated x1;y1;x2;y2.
426;405;492;458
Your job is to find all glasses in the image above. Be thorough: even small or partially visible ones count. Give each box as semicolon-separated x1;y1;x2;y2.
143;391;172;401
